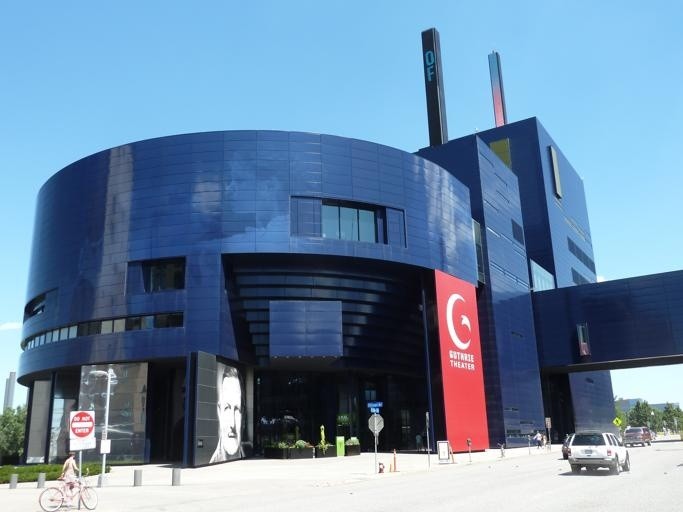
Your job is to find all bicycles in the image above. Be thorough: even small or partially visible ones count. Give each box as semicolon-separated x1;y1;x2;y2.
39;468;99;512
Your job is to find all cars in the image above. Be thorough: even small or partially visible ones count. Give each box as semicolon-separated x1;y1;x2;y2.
561;433;575;460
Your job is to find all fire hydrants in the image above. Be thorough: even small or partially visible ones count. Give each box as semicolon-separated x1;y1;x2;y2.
378;462;385;473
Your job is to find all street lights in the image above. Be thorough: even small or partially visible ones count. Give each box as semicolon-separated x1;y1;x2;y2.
89;367;119;486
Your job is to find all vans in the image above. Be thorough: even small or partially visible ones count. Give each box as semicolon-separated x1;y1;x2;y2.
621;426;652;448
566;432;630;475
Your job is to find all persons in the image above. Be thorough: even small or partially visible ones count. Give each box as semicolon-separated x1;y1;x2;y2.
533;430;547;449
208;363;246;465
415;433;423;452
58;451;80;507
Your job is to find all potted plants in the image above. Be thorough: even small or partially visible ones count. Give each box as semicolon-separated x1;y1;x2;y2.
337;416;350;441
263;436;361;459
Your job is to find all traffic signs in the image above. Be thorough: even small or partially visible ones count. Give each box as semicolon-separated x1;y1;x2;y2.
371;408;380;413
367;401;383;408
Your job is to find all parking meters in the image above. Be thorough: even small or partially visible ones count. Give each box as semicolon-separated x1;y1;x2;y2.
466;439;471;462
